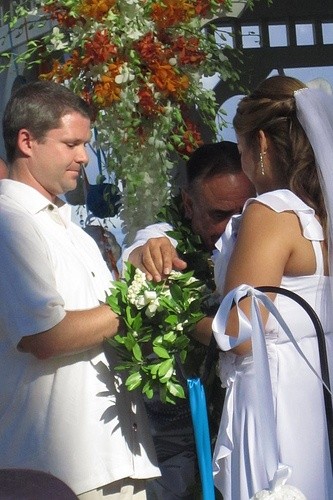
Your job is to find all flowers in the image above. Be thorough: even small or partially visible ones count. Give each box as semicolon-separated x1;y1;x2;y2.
98;261;212;405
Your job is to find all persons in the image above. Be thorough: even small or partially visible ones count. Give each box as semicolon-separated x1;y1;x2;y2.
0;79;163;500
184;74;333;500
118;139;259;500
